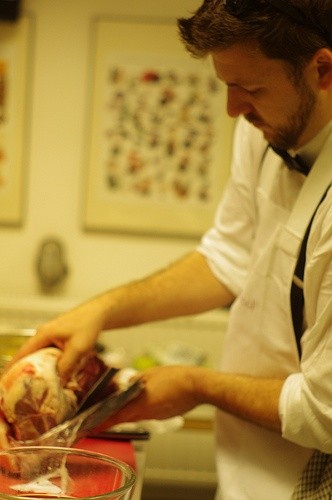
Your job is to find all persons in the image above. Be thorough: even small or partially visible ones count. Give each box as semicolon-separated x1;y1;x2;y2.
1;0;332;500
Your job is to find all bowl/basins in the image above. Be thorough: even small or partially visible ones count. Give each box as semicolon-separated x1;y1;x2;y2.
0;446;137;500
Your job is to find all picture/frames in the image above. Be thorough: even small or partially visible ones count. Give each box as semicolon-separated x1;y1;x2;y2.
80;12;237;244
2;8;35;228
